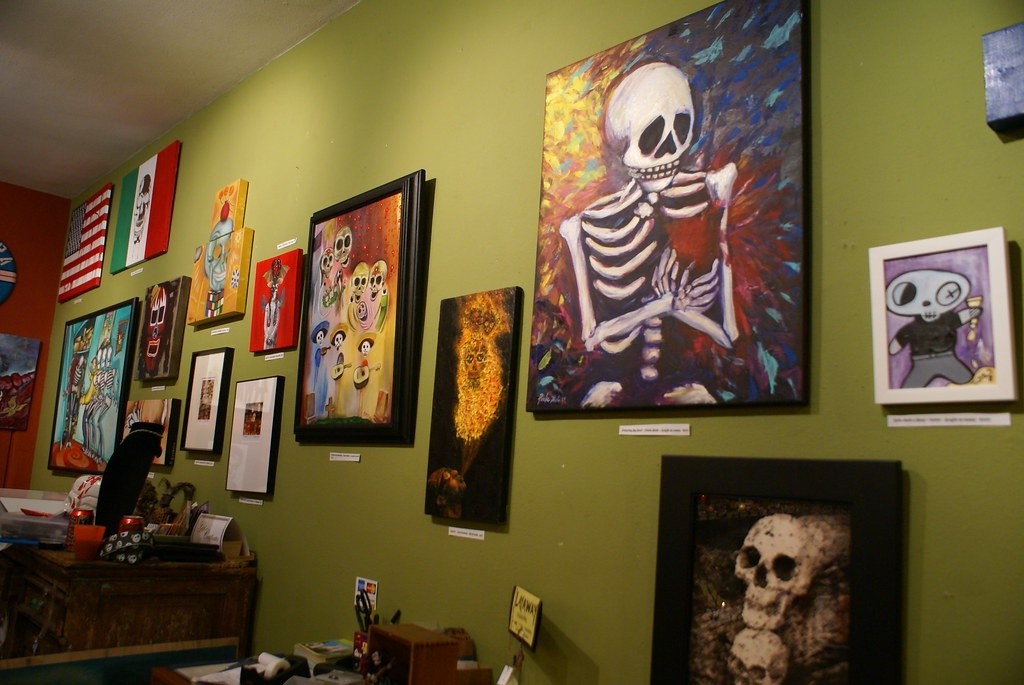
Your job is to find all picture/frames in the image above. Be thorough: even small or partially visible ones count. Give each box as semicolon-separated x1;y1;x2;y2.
178;346;235;453
867;226;1019;410
47;297;140;477
647;454;908;684
292;169;426;447
224;373;285;501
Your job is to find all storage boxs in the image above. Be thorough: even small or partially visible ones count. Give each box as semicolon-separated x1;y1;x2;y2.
364;623;460;685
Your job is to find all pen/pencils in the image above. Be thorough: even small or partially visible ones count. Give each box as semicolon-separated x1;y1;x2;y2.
355;605;365;632
374;614;379;625
389;610;401;625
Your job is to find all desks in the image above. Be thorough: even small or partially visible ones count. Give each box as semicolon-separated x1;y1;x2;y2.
0;547;259;664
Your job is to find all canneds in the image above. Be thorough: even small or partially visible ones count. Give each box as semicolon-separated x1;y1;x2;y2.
66;508;94;554
119;515;146;533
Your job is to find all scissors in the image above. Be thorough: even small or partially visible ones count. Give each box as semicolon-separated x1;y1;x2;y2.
356;588;372;625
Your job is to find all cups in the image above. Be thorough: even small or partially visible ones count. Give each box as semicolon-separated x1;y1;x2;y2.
73;523;106;562
